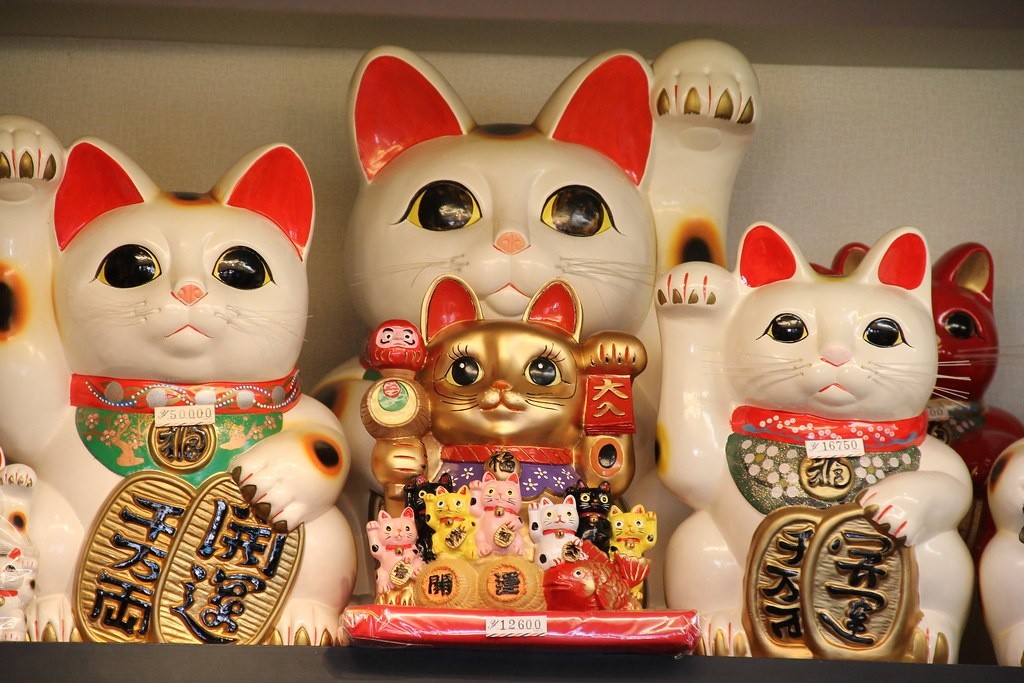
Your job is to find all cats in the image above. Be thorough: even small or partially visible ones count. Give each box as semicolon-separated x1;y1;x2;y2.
2;114;359;649
303;39;1022;669
